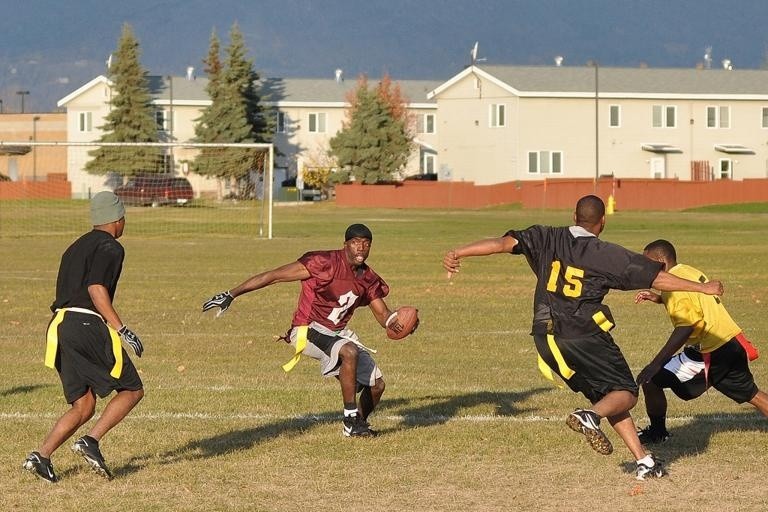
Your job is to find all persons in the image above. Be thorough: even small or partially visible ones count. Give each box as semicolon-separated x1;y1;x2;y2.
442;195;724;482
22;192;144;482
203;223;394;438
636;239;767;447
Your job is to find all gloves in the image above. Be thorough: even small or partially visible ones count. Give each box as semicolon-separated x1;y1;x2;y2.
118;326;144;357
202;291;234;318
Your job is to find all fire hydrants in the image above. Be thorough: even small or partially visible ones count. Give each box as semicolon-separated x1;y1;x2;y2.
605;194;616;214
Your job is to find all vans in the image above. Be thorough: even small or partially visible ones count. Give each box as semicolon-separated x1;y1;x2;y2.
113;174;195;208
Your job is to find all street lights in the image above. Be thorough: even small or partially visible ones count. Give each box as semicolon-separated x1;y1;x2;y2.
167;76;172;176
33;116;40;181
586;57;600;198
16;91;32;114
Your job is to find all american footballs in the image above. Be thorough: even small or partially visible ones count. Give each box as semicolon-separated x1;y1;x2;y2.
386;307;417;340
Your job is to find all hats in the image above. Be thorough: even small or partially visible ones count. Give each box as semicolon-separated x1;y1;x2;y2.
89;191;126;226
344;222;373;243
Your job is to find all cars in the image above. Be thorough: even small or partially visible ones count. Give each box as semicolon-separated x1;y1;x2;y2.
363;177;402;187
403;173;437;183
281;171;347;200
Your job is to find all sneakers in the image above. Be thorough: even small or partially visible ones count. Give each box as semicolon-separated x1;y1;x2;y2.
631;462;670;483
41;458;59;483
636;426;672;447
341;416;383;438
72;436;113;481
567;406;614;455
